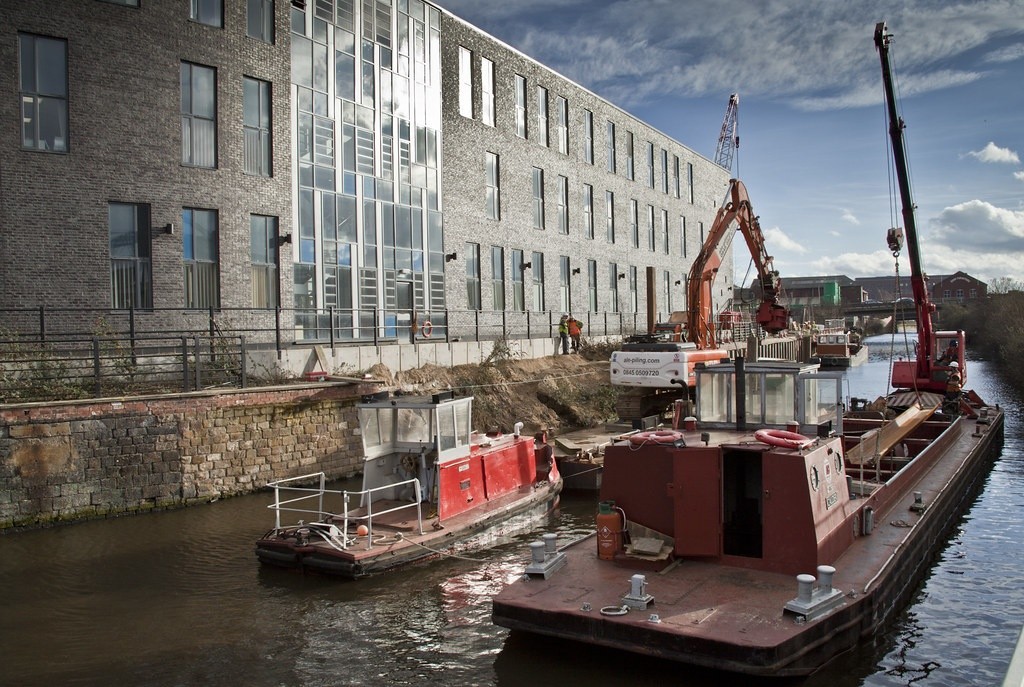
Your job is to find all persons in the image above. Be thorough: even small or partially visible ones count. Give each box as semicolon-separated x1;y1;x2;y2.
567;315;583;354
935;340;959;365
559;313;569;356
945;361;962;401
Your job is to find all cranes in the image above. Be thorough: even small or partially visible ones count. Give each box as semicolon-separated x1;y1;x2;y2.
712;92;741;172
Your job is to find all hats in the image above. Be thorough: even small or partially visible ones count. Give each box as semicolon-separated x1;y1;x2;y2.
564;313;568;316
570;315;576;319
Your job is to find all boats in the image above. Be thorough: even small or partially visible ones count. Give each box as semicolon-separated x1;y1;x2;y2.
254;389;564;581
810;327;869;367
490;335;1004;687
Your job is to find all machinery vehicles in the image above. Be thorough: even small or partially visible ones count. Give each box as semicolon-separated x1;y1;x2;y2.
873;19;966;427
609;177;791;432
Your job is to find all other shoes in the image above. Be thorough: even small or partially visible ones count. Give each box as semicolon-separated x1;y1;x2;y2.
562;350;579;355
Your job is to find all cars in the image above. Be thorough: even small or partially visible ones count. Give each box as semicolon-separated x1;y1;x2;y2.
865;299;883;305
892;297;914;303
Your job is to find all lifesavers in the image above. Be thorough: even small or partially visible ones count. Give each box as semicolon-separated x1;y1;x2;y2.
630;430;685;445
755;428;813;450
422;321;433;338
